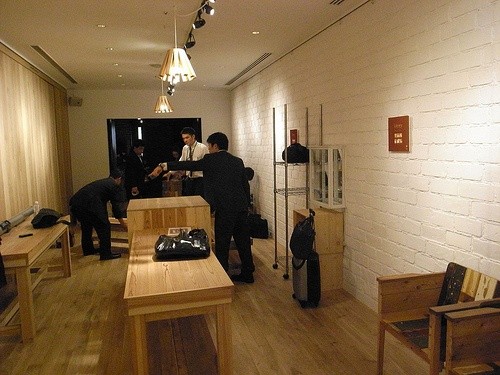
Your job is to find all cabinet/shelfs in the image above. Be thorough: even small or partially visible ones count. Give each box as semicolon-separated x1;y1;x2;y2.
273;104;323;280
293;208;344;291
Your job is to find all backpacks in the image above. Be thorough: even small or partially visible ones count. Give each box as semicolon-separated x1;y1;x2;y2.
31;208;70;228
289;217;315;258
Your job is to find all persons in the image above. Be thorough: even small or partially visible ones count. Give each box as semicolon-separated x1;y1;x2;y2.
149;132;255;283
69;168;127;260
163;127;209;196
123;139;146;199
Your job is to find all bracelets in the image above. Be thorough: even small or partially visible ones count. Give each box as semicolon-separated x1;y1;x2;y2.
158;162;163;169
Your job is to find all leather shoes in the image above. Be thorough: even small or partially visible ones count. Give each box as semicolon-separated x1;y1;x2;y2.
101;252;121;260
93;248;99;253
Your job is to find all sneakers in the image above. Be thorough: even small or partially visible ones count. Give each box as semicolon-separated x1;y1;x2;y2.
230;273;254;283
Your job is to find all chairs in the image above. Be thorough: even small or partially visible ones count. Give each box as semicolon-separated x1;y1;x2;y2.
375;262;500;375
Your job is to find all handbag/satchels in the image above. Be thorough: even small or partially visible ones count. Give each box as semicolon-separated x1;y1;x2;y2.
154;228;210;259
248;214;268;238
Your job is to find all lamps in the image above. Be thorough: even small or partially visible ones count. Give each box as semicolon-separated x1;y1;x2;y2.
167;85;175;91
184;32;195;48
157;6;197;83
168;91;174;96
154;77;174;113
201;2;215;16
192;15;205;29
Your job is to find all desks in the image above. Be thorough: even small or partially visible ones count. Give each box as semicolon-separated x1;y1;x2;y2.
0;215;72;345
124;228;234;375
127;196;212;248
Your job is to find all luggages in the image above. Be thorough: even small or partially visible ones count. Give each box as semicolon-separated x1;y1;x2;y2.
292;209;320;308
